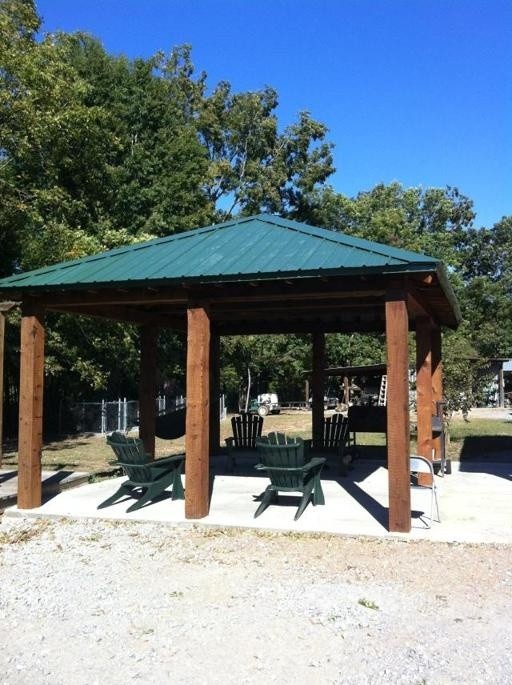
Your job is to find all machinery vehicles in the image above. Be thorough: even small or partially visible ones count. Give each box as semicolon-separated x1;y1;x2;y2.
247;399;281;417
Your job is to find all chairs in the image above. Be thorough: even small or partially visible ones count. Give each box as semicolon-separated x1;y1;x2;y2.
94;430;187;513
311;412;359;475
253;430;330;522
409;456;441;529
223;411;265;473
